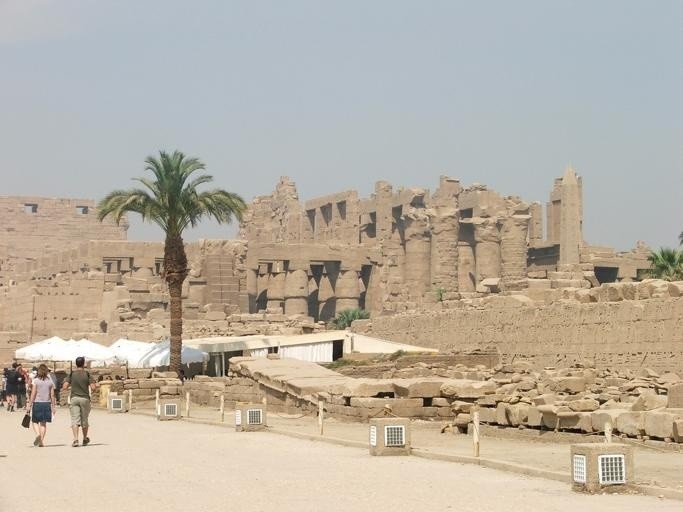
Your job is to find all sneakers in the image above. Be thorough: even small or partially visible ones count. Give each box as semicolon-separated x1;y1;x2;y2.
83;438;89;445
7;403;13;411
72;440;78;446
34;435;43;447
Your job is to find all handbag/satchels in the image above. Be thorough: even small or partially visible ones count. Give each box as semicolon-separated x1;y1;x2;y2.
22;415;31;428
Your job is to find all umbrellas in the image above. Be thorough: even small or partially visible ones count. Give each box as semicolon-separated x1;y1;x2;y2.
15;336;210;381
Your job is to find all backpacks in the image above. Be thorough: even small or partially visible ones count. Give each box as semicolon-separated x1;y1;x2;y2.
8;370;16;383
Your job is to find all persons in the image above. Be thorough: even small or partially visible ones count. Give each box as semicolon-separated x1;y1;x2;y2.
27;364;56;447
61;356;97;448
0;362;58;412
